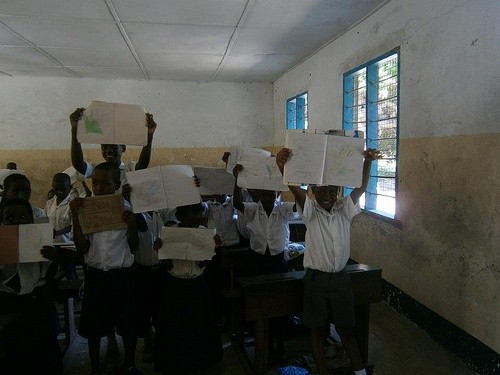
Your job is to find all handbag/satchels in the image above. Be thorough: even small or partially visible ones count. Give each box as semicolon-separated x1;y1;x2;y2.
152;299;224;375
0;309;70;375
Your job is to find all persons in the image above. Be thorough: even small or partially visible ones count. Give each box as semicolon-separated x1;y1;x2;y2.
70;108;157;194
44;173;80;242
70;161;149;375
133;195;243;375
222;152;300;368
0;163;61;375
276;148;382;375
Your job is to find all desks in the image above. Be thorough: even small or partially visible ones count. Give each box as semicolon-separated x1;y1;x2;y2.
40;220;383;375
